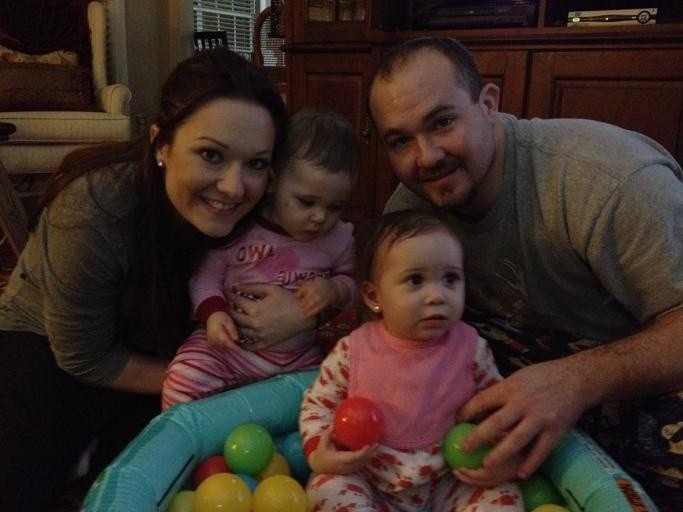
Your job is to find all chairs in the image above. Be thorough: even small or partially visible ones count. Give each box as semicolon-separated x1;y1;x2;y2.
193;30;228;54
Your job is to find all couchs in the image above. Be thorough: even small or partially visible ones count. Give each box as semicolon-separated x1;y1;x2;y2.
0;0;135;175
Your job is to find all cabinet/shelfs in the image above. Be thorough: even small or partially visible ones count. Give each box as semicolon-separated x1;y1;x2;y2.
282;0;683;216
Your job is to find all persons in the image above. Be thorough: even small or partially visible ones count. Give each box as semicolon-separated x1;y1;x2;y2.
160;107;363;413
0;47;292;512
298;208;525;512
363;37;683;481
